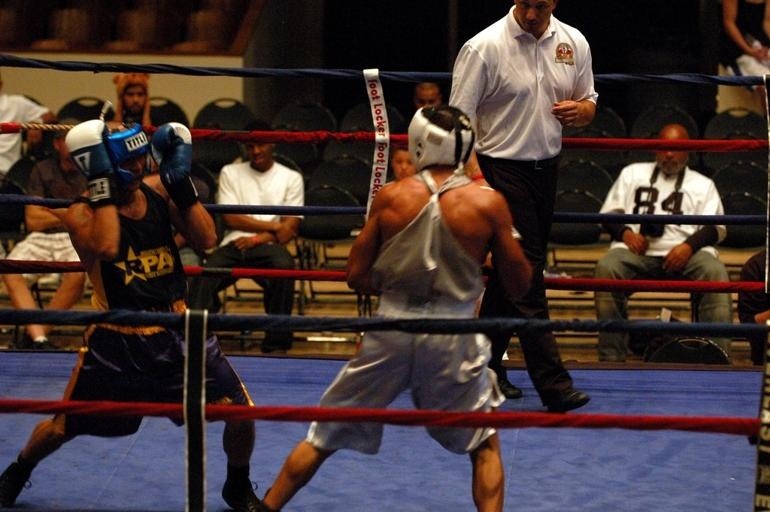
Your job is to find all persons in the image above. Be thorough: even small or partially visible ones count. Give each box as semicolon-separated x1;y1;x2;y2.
738;251;767;363
3;117;86;347
449;0;598;413
595;122;733;363
191;120;305;353
255;108;534;512
112;72;157;131
0;116;270;510
1;80;59;236
392;144;415;180
705;1;770;116
414;82;442;108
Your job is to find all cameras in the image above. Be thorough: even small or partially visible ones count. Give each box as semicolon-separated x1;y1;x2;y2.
639;224;664;238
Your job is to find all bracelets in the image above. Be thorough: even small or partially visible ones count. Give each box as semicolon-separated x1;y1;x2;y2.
471;174;485;183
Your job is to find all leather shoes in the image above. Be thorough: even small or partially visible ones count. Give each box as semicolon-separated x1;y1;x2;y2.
548;388;589;412
499;380;522;399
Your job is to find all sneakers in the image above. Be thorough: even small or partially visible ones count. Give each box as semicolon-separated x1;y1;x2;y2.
0;462;31;512
8;332;60;350
222;480;271;512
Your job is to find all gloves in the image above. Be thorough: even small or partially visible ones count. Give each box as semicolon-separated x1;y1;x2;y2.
149;123;198;209
65;120;118;208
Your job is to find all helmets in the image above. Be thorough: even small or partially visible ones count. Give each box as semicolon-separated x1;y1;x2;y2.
104;122;151;181
408;107;475;173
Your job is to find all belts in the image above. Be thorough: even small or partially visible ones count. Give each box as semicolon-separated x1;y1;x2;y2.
481;156;562;170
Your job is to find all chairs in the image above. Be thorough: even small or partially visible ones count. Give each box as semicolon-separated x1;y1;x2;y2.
0;94;769;363
